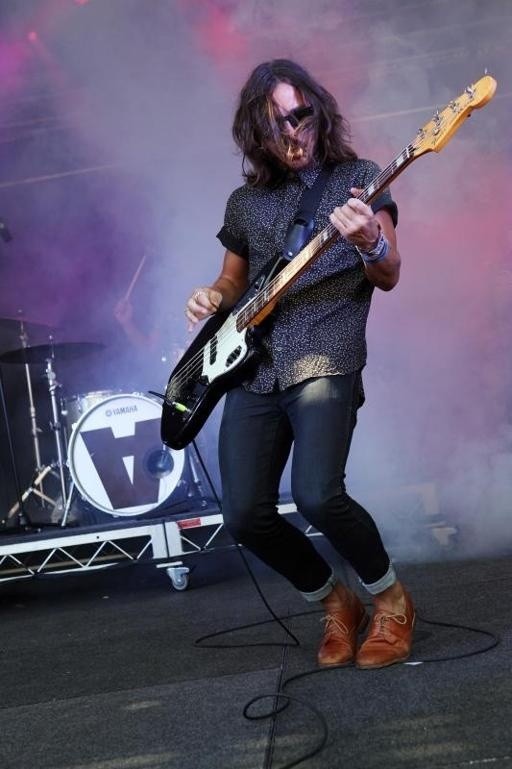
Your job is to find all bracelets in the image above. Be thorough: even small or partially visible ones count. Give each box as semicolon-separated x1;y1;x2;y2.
355;220;390;268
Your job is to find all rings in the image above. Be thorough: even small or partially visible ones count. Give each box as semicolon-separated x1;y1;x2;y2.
193;292;201;298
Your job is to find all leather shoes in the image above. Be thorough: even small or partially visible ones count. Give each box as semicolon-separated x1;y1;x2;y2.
356;592;419;672
316;598;372;670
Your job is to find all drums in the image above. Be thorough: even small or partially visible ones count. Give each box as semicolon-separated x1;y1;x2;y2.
67;393;206;516
63;387;145;453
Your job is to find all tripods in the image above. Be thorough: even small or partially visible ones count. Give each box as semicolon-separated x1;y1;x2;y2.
0;375;84;527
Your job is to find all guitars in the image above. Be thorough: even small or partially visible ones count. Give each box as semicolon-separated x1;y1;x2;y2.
160;76;497;448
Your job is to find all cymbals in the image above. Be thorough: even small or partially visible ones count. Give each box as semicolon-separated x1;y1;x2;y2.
0;342;104;363
3;317;67;339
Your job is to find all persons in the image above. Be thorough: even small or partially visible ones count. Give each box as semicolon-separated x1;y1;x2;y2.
182;56;420;675
114;288;198;382
